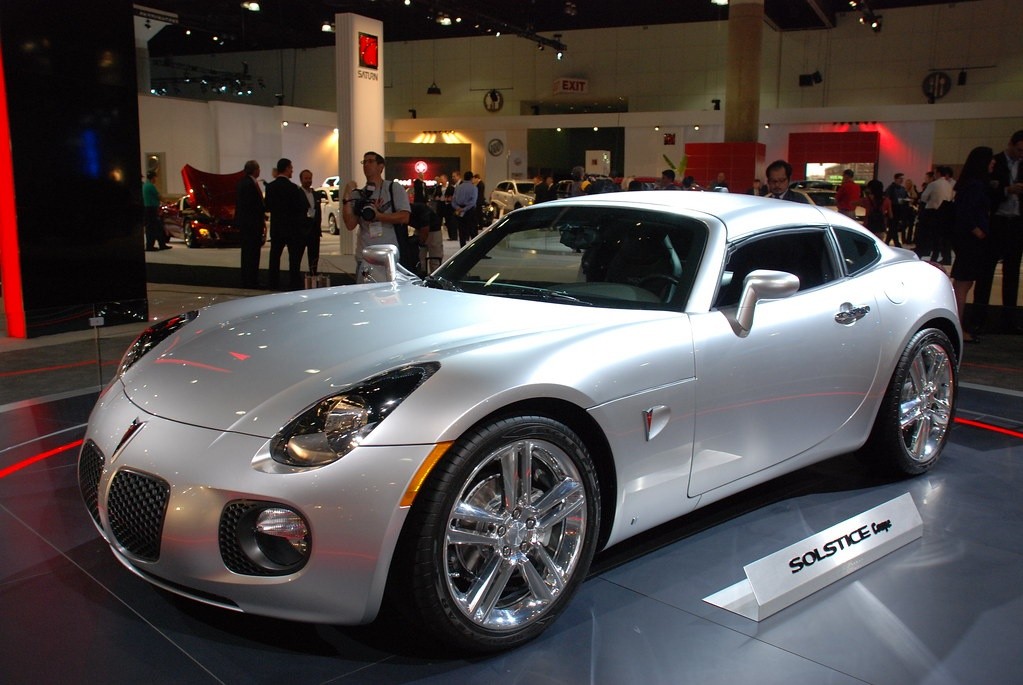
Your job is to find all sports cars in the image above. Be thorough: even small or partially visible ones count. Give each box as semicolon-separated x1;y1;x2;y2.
76;191;964;650
154;164;269;249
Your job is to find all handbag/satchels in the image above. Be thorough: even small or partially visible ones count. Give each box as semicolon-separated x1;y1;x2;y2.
928;199;979;249
398;236;421;274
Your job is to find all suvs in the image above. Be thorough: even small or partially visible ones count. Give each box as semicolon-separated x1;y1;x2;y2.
489;178;536;219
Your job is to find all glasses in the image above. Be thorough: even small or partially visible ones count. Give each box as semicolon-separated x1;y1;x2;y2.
769;175;788;183
360;159;378;164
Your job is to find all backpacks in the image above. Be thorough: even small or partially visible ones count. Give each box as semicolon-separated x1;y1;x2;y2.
865;195;887;232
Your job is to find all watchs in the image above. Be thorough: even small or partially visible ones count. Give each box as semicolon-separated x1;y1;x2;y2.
343;198;352;205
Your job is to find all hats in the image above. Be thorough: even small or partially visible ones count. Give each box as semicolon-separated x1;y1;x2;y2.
147;171;157;177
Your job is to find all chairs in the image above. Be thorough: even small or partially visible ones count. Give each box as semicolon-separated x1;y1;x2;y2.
601;227;682;305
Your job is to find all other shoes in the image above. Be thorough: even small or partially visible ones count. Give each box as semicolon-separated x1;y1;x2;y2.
145;247;159;252
160;245;172;250
937;259;951;265
480;256;492;259
962;335;981;343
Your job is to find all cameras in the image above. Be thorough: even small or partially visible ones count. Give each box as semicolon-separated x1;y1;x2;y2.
342;182;377;222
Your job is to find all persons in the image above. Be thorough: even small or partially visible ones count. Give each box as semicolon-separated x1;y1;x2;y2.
142;170;173;251
343;152;410;274
231;158;321;287
412;169;485;273
492;131;1023;342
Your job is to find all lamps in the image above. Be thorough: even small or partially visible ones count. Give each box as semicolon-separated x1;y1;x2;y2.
151;0;267;99
409;108;417;118
423;129;455;134
593;126;599;131
654;126;660;131
694;125;701;131
427;40;442;95
304;123;309;128
535;41;546;53
557;51;564;60
400;0;501;38
846;0;884;33
556;127;561;132
711;98;721;110
764;123;770;129
321;18;333;33
491;89;498;102
282;121;288;127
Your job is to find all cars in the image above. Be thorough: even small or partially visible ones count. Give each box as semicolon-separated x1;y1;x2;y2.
789;188;866;226
314;186;339;235
481;198;493;226
